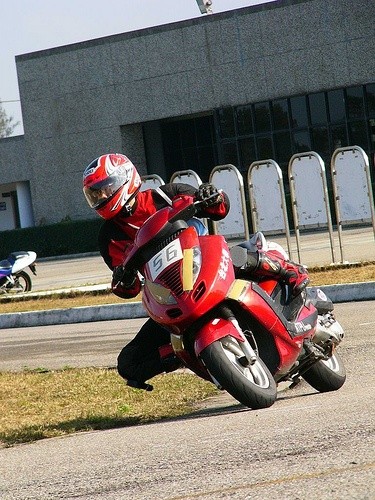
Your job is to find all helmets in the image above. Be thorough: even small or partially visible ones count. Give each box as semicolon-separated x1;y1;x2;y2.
82;154;142;221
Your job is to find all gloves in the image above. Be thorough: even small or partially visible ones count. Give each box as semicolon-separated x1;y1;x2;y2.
111;264;138;287
195;183;219;206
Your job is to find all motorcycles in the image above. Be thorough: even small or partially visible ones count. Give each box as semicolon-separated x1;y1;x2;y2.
112;193;346;409
0;250;37;294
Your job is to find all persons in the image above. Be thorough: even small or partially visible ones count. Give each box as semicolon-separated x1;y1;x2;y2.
82;153;308;381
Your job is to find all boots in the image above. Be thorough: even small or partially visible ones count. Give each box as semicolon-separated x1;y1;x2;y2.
158;343;184;374
252;250;310;296
